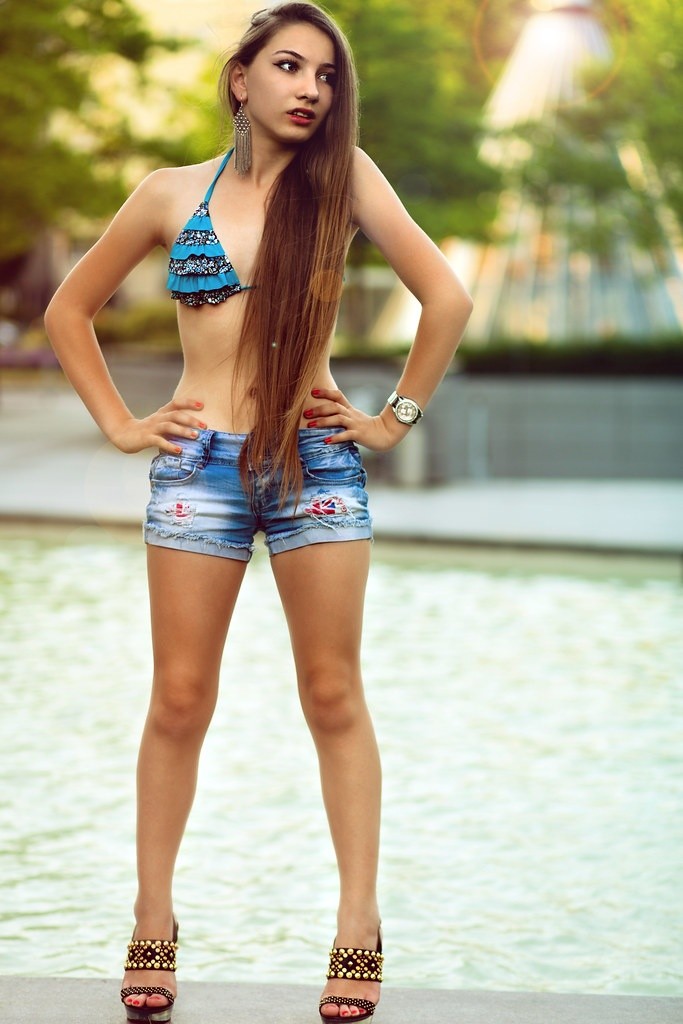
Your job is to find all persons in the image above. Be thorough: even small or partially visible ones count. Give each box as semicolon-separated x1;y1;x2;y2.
43;1;474;1024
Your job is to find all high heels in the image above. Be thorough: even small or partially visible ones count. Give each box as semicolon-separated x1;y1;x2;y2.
121;912;179;1024
319;928;384;1024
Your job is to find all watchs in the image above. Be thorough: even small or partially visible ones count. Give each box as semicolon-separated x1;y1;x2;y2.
386;391;423;426
